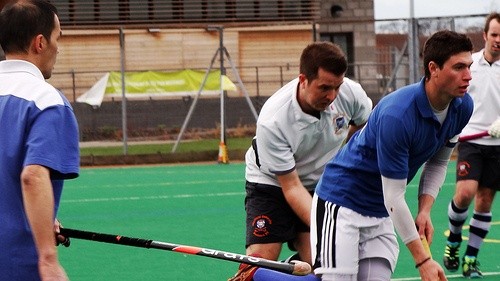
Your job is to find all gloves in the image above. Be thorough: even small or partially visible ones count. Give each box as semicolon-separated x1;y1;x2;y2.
488;117;500;137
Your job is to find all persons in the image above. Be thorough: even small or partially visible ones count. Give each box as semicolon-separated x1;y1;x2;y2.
440;12;500;279
230;41;372;281
0;0;81;281
310;29;475;281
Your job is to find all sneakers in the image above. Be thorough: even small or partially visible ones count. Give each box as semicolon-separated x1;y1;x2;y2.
443;238;461;271
461;256;482;278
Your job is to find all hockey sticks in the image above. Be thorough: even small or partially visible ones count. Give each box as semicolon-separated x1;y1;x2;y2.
60;228;311;277
417;226;433;260
458;129;493;142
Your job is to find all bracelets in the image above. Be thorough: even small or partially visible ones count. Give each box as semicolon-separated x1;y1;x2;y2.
416;257;430;269
